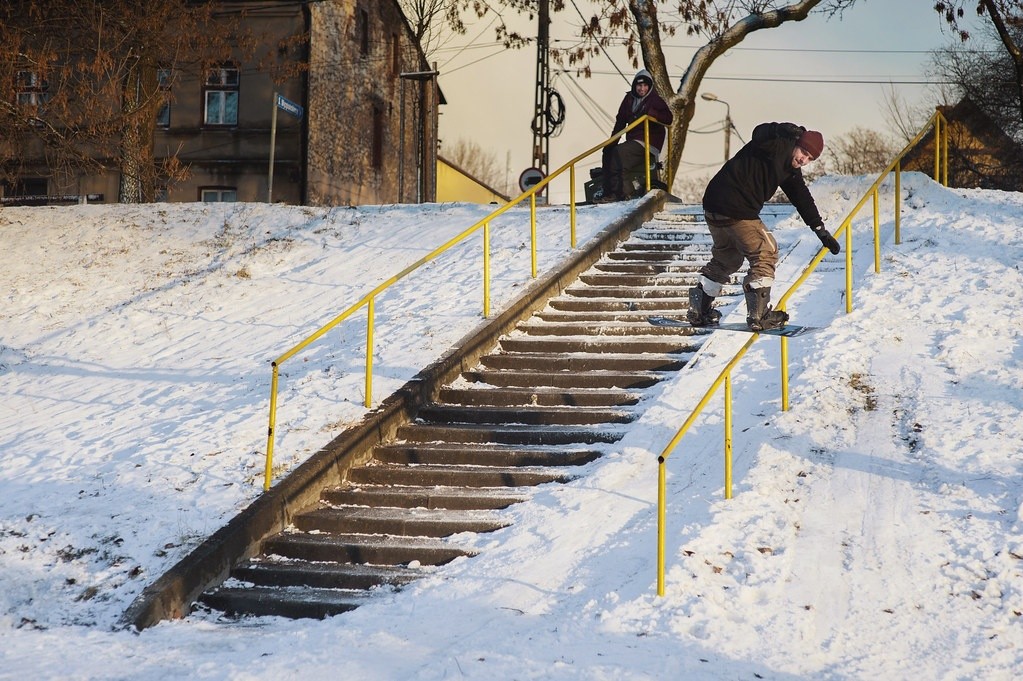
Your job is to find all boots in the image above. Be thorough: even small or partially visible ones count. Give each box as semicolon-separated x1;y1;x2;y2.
686;276;724;327
741;276;790;331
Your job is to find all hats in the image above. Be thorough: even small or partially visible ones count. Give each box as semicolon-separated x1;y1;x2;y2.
797;130;824;162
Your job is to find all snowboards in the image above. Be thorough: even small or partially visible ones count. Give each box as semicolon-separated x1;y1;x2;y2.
647;315;831;338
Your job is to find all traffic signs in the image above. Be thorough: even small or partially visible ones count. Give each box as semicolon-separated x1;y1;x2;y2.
276;94;304;119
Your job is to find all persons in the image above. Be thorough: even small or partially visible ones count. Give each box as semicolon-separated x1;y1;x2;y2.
589;69;672;203
683;122;841;333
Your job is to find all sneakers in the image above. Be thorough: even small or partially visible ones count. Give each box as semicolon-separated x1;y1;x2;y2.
591;190;626;205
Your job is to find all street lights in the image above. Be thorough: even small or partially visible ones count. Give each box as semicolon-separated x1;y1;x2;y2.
700;93;735;162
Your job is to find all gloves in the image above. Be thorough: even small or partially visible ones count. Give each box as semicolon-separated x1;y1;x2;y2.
775;122;806;141
814;224;841;255
603;132;621;153
